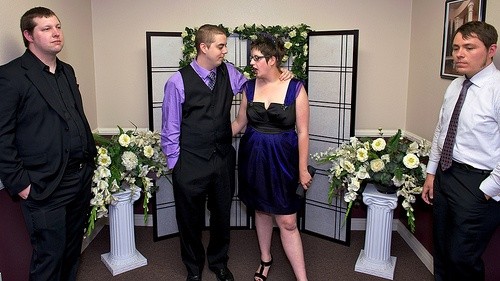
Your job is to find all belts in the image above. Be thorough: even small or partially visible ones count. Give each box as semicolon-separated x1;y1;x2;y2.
473;170;493;174
69;160;86;171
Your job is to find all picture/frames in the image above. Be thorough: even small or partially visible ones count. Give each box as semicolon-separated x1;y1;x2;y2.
439;0;488;81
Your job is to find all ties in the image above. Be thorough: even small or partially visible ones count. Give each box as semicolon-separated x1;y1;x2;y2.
207;71;216;91
439;80;474;172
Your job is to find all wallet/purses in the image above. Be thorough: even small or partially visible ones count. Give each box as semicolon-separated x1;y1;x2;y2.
295;165;317;201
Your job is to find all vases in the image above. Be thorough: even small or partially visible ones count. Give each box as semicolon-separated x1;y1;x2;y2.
374;176;399;195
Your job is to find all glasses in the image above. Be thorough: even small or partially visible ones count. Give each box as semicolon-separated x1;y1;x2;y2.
249;54;265;61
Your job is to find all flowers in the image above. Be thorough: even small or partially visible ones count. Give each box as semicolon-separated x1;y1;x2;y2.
83;123;166;239
176;22;312;82
310;126;433;234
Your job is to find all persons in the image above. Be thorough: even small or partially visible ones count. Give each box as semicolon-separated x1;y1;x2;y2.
420;20;500;281
232;32;314;281
161;24;295;281
0;6;96;281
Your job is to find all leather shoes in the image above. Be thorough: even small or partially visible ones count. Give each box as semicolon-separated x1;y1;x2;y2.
186;272;202;281
208;267;234;281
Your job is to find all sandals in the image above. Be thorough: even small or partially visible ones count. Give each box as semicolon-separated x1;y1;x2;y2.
253;254;274;281
